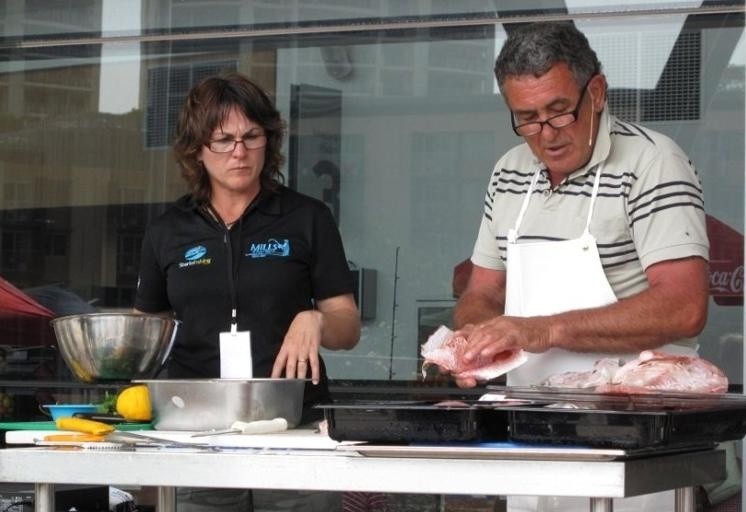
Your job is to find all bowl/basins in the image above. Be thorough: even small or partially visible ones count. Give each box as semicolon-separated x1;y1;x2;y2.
42;404;97;422
47;313;181;383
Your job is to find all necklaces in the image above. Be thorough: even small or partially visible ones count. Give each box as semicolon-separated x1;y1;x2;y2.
224;221;235;226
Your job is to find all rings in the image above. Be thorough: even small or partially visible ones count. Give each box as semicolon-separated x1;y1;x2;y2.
297;358;309;363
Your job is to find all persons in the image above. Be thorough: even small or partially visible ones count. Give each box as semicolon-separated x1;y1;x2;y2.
133;73;360;511
436;23;712;512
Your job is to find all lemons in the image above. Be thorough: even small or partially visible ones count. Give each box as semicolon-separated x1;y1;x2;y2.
116;385;152;421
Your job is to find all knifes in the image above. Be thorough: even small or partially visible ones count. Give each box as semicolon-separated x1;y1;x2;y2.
45;417;287;451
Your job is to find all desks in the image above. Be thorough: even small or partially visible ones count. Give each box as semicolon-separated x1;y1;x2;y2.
0;415;728;510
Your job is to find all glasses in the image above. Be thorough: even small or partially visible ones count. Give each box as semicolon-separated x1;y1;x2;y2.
511;73;596;137
203;131;269;153
